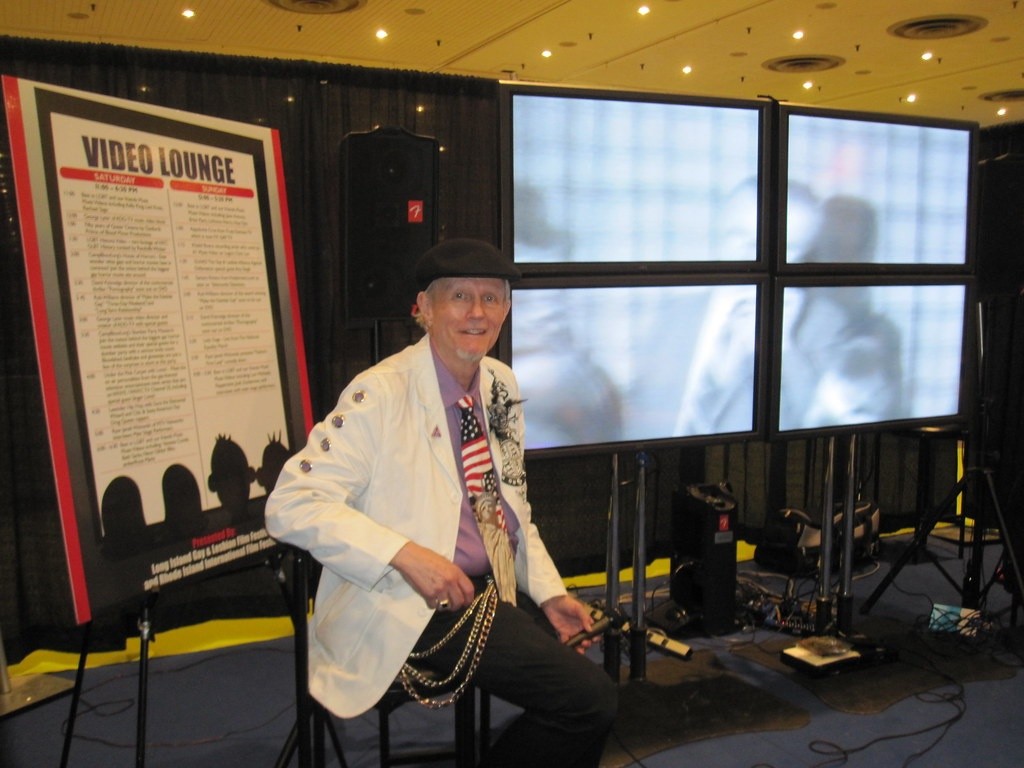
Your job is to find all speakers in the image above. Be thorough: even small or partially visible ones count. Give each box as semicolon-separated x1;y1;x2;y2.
979;153;1024;298
341;125;442;327
667;482;738;636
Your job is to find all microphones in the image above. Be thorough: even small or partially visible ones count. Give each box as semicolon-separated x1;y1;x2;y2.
566;606;628;648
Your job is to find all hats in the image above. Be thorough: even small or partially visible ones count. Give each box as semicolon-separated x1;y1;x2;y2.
416;239;521;291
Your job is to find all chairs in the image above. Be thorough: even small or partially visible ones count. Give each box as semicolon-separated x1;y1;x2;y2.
874;425;971;561
294;545;490;768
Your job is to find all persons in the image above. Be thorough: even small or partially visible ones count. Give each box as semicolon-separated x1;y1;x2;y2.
265;238;618;768
511;175;904;446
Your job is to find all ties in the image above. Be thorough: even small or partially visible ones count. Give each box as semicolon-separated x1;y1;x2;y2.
457;394;517;606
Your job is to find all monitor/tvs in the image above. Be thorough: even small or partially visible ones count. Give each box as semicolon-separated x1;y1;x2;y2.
495;79;980;460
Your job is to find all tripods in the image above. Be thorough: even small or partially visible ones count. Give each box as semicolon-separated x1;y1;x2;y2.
861;415;1024;618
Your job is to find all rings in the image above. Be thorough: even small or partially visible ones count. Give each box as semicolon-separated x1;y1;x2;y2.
440;600;449;608
